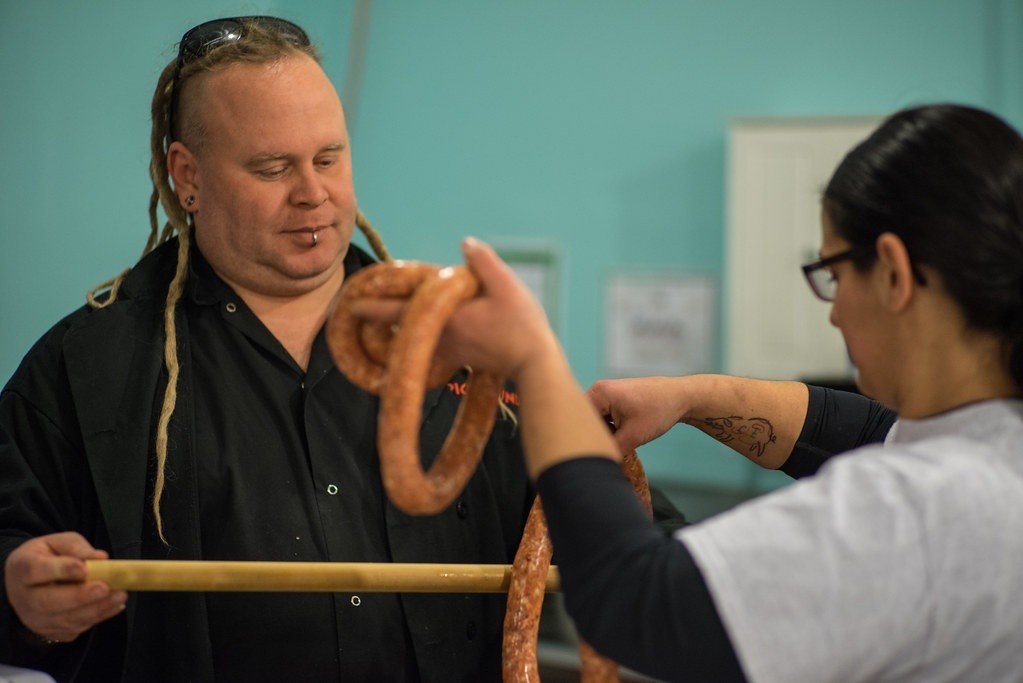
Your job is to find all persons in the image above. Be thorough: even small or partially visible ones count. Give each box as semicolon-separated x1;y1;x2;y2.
353;103;1023;683
0;16;685;683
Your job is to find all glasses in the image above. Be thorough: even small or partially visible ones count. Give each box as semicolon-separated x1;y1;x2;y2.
168;15;310;144
802;243;929;302
